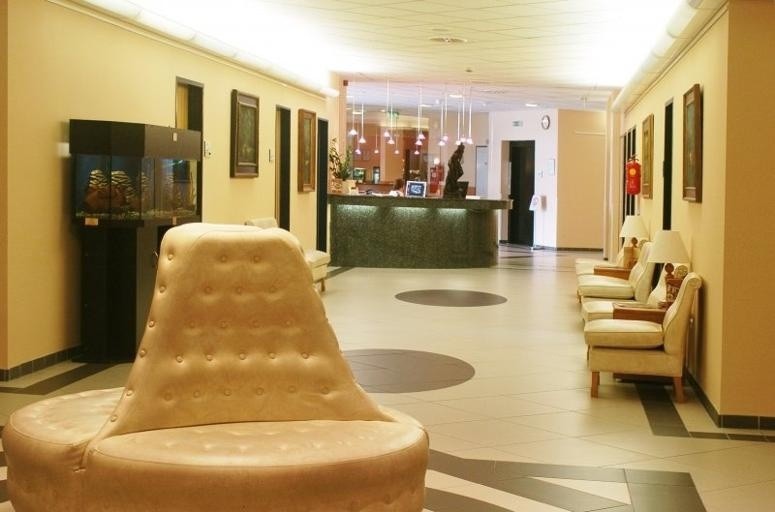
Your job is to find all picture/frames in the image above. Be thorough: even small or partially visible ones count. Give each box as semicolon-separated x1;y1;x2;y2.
683;83;703;203
230;89;259;179
298;108;316;193
641;113;655;199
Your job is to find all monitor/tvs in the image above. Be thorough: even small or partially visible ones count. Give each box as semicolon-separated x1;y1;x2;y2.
456;181;469;196
406;181;426;197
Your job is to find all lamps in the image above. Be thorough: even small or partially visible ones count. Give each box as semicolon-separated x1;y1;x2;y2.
618;215;649;248
646;230;690;306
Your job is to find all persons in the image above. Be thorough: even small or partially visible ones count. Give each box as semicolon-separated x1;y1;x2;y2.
388;177;405;196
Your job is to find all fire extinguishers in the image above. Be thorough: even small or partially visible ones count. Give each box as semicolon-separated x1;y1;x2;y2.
625;154;641;194
429;166;439;193
437;163;445;182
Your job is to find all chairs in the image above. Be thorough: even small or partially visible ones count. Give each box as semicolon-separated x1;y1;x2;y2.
243;214;332;292
576;237;701;403
